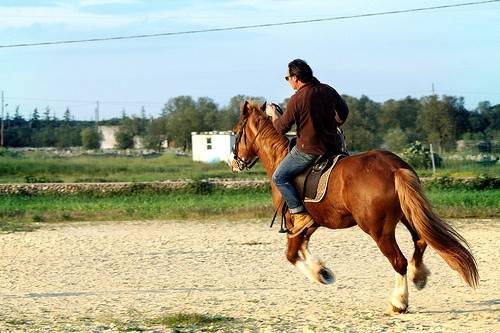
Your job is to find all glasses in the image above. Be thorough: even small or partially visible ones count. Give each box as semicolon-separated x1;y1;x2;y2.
285;75;294;81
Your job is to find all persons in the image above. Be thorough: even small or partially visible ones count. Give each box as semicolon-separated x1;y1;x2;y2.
265;59;350;239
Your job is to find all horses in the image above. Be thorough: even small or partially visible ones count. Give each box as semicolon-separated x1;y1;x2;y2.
225;100;480;316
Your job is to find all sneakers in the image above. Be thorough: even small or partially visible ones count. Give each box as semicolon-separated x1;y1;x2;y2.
288;212;314;238
